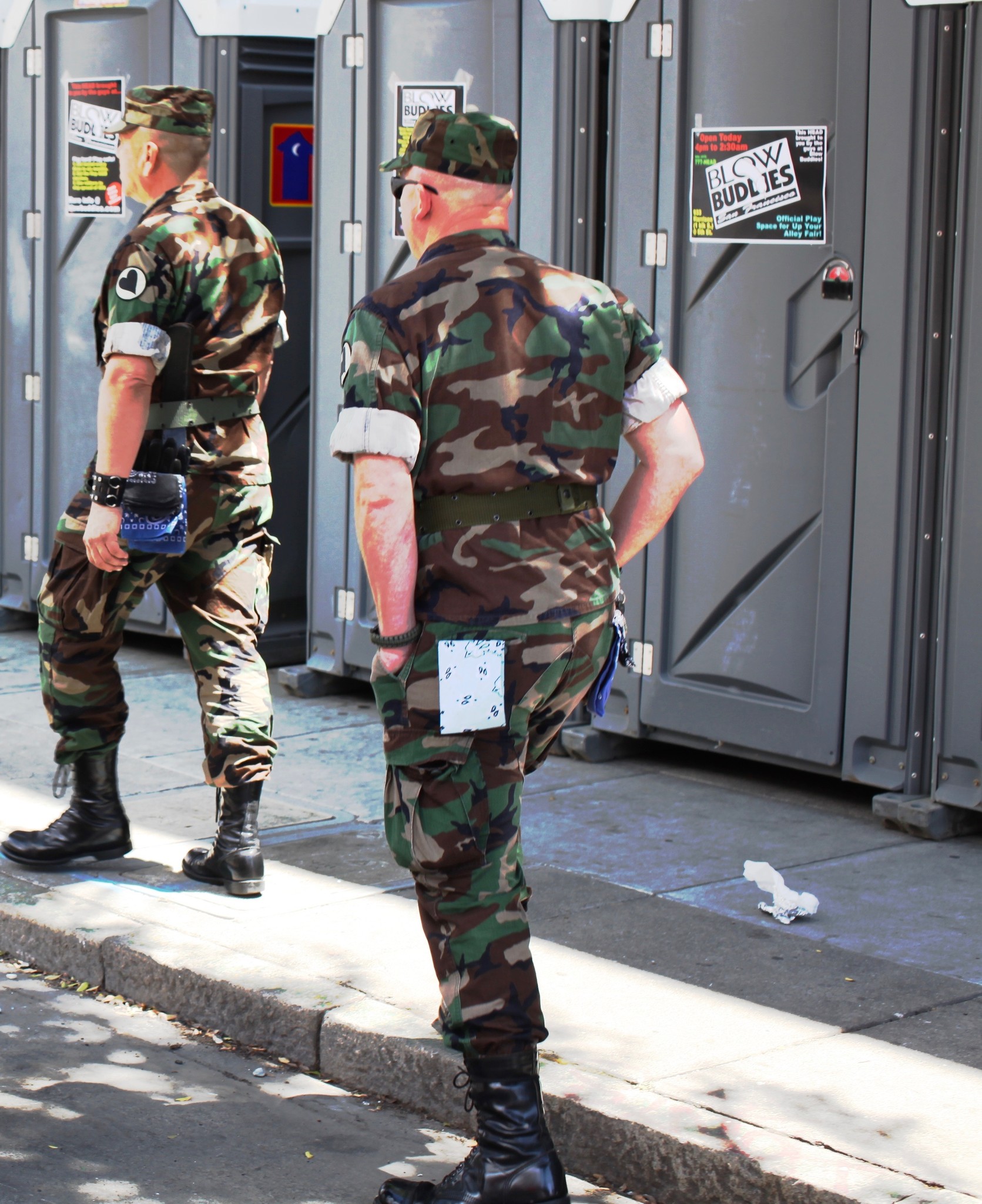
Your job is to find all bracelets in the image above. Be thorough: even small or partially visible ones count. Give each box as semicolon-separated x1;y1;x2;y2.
91;471;128;507
370;621;423;648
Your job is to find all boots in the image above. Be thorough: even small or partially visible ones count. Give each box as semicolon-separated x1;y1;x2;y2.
0;743;133;865
182;778;265;896
376;1069;572;1204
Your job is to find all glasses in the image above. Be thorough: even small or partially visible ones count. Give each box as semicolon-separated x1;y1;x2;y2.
390;175;439;198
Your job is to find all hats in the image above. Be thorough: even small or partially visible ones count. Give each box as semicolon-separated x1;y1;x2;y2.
378;107;518;185
103;84;215;138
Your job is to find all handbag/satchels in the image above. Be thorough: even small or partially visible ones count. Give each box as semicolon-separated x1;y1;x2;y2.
119;473;188;555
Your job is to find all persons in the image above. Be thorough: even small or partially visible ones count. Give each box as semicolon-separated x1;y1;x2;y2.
0;84;286;898
331;109;704;1204
